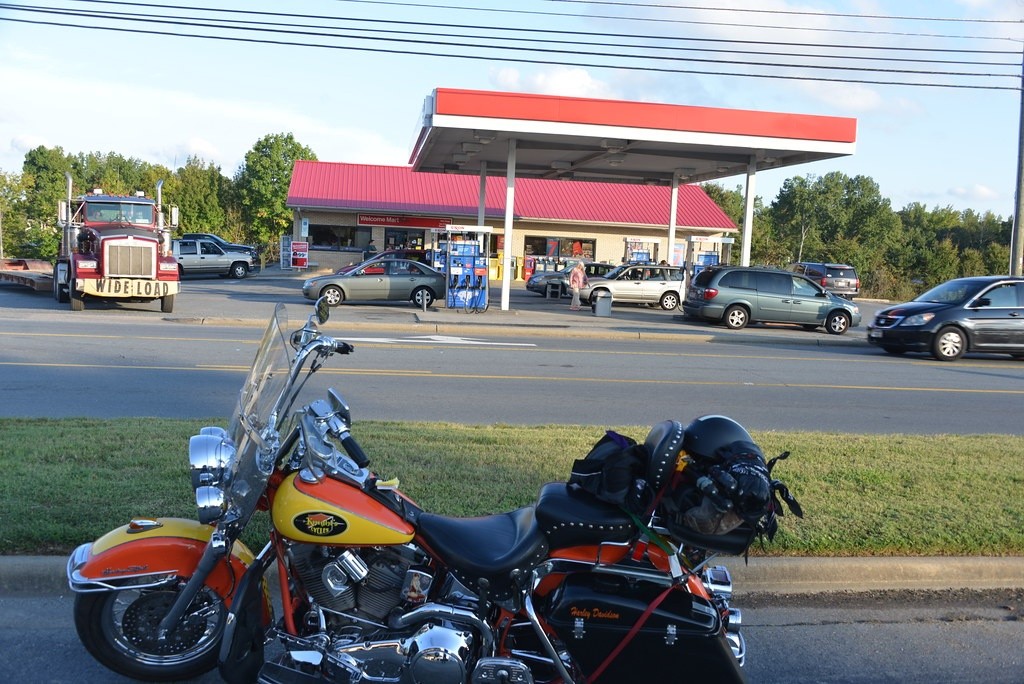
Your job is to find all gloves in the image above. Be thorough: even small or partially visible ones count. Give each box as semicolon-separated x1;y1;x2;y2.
695;441;772;517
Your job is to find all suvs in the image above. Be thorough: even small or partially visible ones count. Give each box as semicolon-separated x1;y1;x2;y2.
580;263;686;311
795;262;860;301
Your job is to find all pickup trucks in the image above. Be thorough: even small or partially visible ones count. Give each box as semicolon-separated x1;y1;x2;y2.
170;233;256;279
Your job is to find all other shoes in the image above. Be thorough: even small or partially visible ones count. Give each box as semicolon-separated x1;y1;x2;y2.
570;306;583;311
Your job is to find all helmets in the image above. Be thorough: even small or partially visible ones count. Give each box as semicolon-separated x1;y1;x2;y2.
682;417;750;467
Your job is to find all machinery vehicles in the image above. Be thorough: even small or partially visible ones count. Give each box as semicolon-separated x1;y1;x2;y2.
53;171;181;313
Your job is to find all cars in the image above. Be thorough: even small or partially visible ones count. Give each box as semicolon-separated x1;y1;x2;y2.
336;249;432;275
302;258;446;308
527;262;617;296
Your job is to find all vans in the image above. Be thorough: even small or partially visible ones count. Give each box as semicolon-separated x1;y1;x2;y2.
683;265;861;335
868;276;1024;361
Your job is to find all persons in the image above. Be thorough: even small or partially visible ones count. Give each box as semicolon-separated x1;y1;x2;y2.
569;260;591;311
367;240;378;258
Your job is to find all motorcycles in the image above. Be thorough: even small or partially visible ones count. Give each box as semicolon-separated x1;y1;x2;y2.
67;296;804;684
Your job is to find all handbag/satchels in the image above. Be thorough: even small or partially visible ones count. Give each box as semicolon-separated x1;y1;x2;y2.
567;430;654;516
